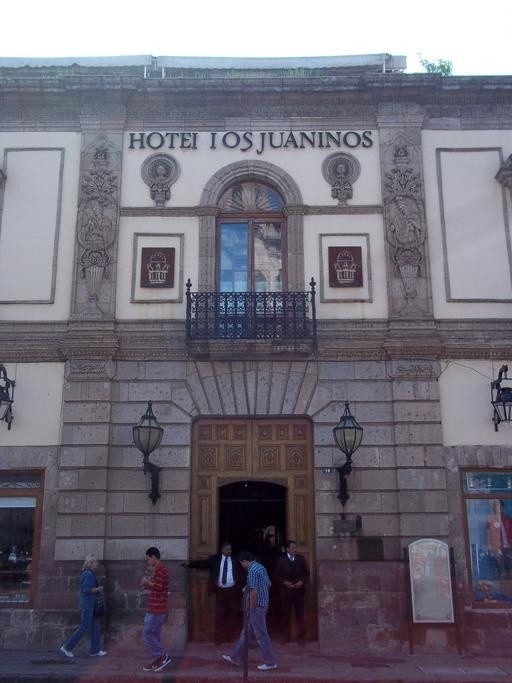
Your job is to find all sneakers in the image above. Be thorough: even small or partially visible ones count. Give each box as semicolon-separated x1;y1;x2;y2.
143;661;169;671
155;652;171;672
257;663;277;671
60;646;74;658
90;650;107;657
222;654;243;666
218;587;235;591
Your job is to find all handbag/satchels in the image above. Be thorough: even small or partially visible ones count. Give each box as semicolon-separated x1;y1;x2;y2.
95;596;105;618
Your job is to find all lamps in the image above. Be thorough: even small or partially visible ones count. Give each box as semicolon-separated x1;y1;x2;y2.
131;398;164;505
0;363;16;430
332;398;365;506
490;363;512;432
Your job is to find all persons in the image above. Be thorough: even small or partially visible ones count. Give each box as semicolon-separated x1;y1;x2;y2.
277;539;308;646
59;554;106;657
140;548;172;670
485;498;512;592
222;548;278;669
179;541;248;646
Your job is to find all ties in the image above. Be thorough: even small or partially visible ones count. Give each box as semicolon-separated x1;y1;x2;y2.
222;556;229;584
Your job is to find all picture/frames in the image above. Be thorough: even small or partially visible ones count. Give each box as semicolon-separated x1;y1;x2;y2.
129;231;185;304
317;232;374;303
